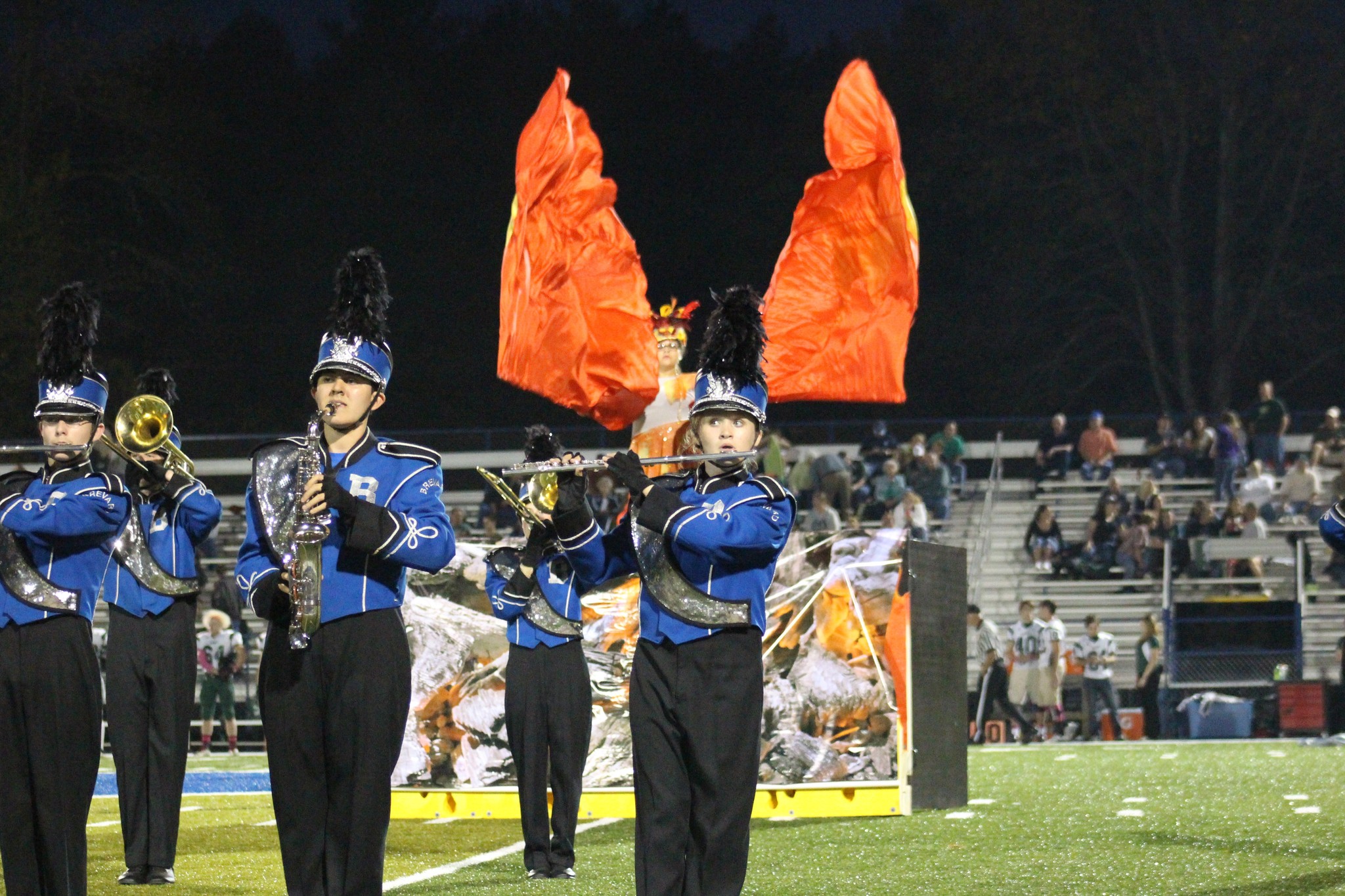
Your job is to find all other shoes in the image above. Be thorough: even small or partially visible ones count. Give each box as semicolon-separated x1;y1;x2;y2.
229;748;239;756
968;739;983;746
195;749;210;757
1022;730;1038;745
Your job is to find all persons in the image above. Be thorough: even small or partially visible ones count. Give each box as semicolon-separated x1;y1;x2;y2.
549;374;795;896
236;332;455;896
0;371;133;896
483;484;592;878
102;426;221;884
0;305;1345;784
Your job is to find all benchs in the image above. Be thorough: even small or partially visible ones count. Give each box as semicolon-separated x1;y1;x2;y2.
925;466;1345;695
91;483;484;762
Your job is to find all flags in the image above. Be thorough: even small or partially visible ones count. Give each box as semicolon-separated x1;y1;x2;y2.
758;58;919;401
496;67;659;431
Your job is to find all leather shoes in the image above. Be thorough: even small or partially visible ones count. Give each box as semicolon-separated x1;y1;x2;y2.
118;866;147;884
527;869;551;879
147;866;175;884
551;867;576;879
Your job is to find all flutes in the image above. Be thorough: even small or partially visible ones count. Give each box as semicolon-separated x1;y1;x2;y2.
502;449;757;477
0;443;88;455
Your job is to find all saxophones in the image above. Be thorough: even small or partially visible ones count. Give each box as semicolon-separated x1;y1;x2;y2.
289;402;338;650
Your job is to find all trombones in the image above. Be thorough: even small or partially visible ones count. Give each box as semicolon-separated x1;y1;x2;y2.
94;395;197;486
472;463;575;555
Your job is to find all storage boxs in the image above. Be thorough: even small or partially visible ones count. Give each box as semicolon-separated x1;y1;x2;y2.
1181;699;1256;740
1101;705;1146;741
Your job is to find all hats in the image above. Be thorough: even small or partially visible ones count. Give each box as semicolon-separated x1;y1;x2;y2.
966;604;980;613
309;245;393;394
515;426;562;524
137;369;182;454
651;299;700;347
689;284;768;425
33;282;109;424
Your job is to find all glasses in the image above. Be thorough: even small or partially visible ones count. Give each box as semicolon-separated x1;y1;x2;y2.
656;342;681;350
